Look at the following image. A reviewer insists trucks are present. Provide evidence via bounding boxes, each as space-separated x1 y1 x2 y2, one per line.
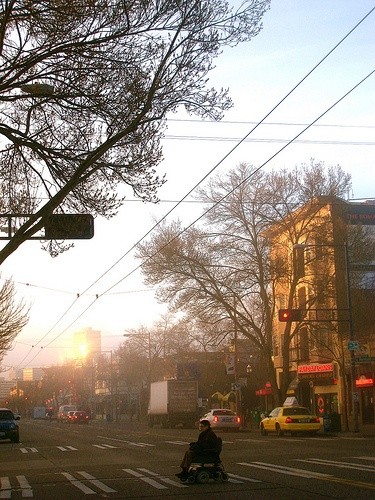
147 378 198 428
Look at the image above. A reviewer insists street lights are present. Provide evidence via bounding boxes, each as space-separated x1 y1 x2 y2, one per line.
13 84 54 94
91 350 114 422
123 333 151 385
198 293 241 416
294 241 361 433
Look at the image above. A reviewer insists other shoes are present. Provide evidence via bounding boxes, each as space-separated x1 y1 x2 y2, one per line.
176 470 190 478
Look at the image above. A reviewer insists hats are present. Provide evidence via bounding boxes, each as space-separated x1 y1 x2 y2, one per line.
200 420 210 426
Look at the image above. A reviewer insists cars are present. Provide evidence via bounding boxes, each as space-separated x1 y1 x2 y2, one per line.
260 397 321 437
0 408 20 443
33 404 89 425
200 409 241 432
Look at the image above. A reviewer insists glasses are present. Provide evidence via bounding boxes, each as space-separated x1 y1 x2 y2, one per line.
200 425 207 428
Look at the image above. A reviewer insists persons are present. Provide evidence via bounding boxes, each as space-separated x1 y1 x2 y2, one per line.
175 420 218 482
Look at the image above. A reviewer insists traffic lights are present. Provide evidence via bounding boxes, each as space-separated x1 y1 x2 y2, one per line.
278 309 302 322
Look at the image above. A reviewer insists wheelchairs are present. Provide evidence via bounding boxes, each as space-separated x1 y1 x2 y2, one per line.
174 437 229 484
